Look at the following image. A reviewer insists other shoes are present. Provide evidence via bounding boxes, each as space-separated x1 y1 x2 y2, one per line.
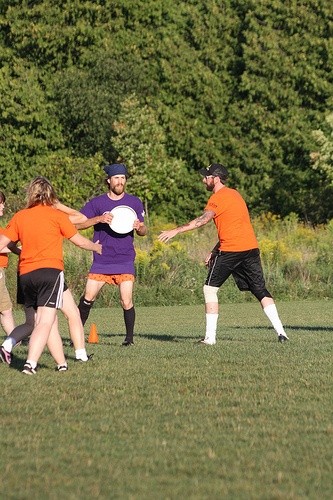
0 345 13 367
57 362 68 372
195 339 212 347
278 332 289 344
74 354 94 362
123 339 134 346
22 362 38 375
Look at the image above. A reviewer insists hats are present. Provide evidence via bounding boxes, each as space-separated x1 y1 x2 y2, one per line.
199 164 228 180
108 164 127 177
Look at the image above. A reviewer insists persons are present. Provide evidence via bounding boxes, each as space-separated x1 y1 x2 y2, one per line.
0 178 103 376
69 163 146 349
158 163 290 345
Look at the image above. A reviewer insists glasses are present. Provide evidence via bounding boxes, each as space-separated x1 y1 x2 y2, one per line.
205 175 215 179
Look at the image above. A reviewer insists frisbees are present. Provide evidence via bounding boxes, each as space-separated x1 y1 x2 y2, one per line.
107 204 138 235
